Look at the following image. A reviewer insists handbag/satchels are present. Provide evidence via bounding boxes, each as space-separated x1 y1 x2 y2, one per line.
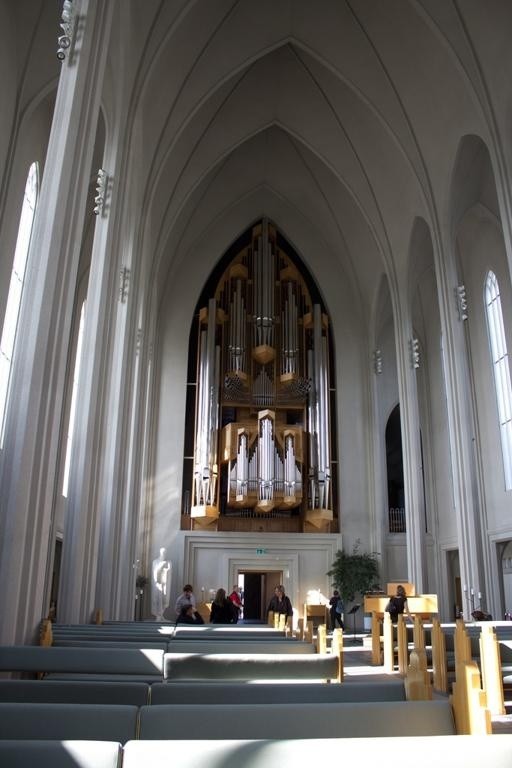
386 602 396 612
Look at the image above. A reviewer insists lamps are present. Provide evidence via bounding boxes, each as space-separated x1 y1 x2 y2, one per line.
90 169 108 216
55 1 74 62
411 337 422 369
454 283 469 322
191 221 335 530
373 348 383 376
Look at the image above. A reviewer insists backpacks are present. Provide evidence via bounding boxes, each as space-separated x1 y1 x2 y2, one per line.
336 599 344 614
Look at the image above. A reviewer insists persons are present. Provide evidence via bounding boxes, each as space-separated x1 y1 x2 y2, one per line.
329 590 345 633
174 584 244 625
385 585 415 627
267 585 293 621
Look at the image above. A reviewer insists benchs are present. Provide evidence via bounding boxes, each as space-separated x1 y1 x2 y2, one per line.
0 607 512 768
